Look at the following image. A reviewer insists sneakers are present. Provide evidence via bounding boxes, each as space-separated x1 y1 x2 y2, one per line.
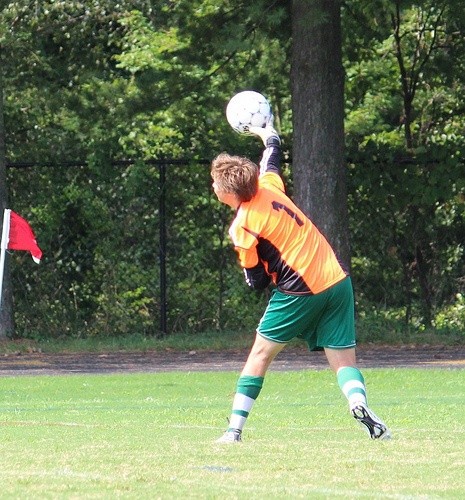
349 402 388 440
216 429 242 444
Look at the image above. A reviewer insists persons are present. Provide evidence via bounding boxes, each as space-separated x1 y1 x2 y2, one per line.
211 113 390 444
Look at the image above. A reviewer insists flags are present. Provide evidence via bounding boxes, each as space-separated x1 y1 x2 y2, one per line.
1 209 42 264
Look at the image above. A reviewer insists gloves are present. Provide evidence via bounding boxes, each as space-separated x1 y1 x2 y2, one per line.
249 114 283 146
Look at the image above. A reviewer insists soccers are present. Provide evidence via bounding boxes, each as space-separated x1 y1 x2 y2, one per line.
226 91 270 137
